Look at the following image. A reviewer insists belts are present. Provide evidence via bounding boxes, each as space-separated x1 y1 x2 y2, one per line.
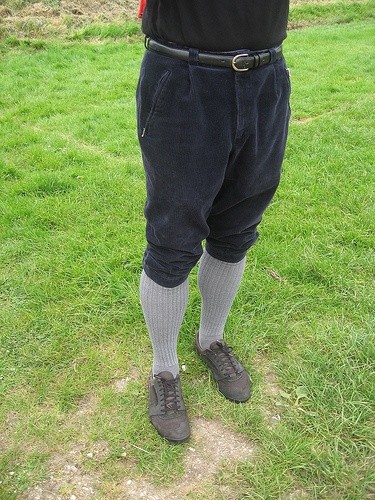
147 38 283 71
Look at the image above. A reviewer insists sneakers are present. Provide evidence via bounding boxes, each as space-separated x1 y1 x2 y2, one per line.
147 371 192 442
193 332 252 403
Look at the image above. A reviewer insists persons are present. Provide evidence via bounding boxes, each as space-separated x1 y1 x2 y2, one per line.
132 1 294 446
